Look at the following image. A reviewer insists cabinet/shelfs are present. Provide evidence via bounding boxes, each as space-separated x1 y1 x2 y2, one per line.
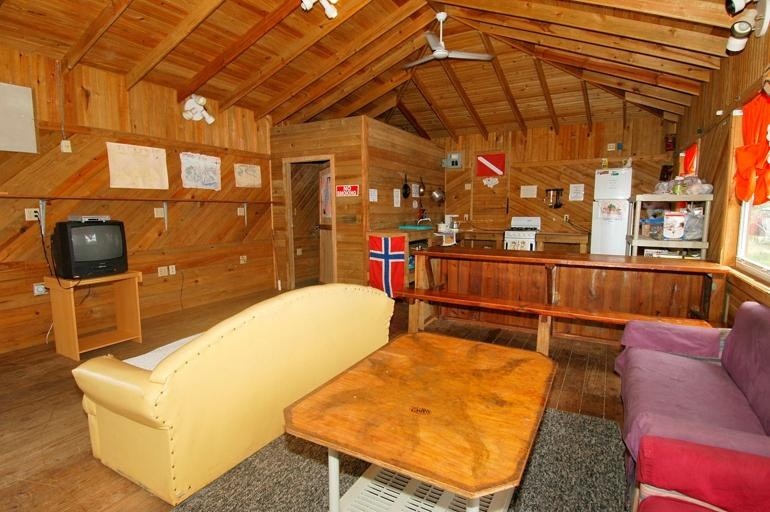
626 194 714 261
399 226 434 288
43 271 143 362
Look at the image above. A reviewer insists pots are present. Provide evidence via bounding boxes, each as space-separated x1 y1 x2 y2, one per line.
403 175 410 198
418 176 425 195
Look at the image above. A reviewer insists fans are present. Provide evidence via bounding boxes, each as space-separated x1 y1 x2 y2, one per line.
403 12 495 68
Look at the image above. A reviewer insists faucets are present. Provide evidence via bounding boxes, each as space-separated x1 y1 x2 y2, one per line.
417 218 431 224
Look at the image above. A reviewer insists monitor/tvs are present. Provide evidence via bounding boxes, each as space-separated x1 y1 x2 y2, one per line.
51 221 128 280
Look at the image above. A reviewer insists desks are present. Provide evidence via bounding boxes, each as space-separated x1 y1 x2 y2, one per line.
459 230 504 249
535 231 589 253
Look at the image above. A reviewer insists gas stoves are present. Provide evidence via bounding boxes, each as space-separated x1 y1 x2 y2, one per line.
505 227 537 231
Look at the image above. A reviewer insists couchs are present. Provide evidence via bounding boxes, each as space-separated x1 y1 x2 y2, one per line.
614 300 770 483
70 282 395 507
631 435 770 512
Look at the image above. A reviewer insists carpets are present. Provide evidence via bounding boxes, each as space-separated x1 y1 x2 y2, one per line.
168 407 629 512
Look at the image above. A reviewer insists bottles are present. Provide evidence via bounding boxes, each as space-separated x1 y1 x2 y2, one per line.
674 176 683 194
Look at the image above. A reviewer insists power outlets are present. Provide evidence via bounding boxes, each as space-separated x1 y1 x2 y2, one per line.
464 214 468 221
33 283 48 295
564 215 569 222
25 208 39 221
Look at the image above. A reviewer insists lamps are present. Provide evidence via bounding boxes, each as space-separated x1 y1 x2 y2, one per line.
725 0 770 56
301 0 338 19
182 93 215 124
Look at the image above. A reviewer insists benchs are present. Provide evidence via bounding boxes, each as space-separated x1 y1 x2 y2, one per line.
392 288 713 357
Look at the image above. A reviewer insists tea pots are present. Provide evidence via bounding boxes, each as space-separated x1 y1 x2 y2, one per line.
449 220 460 229
